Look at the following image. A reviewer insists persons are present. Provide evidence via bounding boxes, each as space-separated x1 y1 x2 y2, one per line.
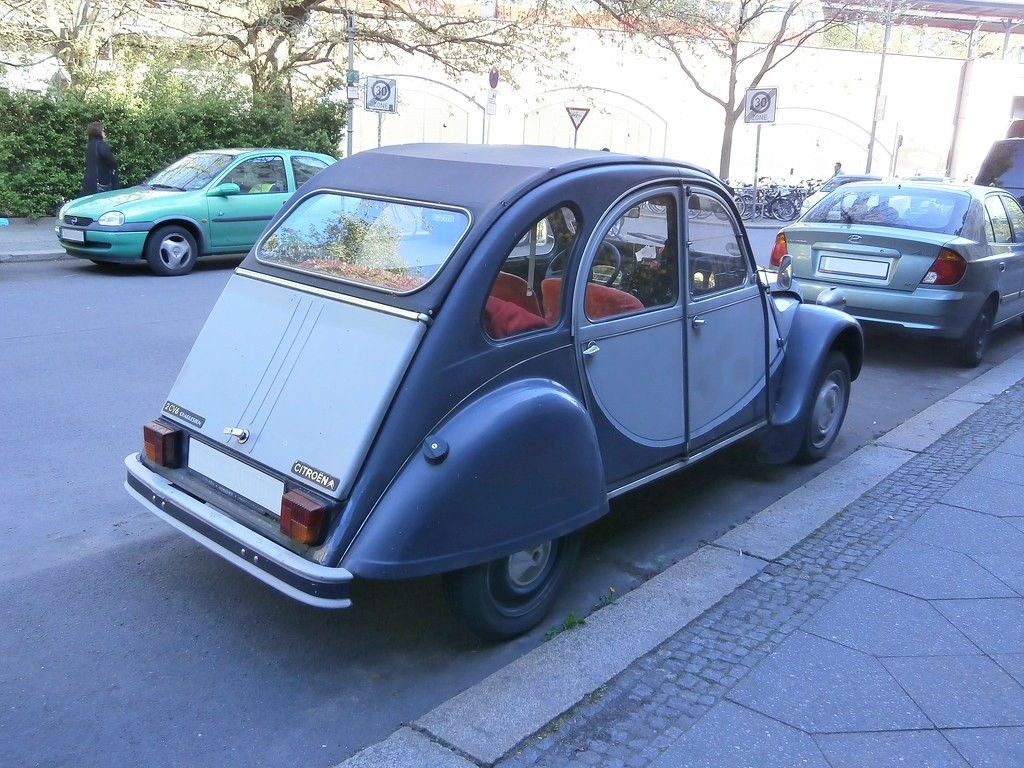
82 122 118 194
834 162 844 175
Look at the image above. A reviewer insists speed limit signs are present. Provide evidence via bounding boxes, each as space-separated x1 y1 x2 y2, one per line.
364 75 398 114
744 88 777 122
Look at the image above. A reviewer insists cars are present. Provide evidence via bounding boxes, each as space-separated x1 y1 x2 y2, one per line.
768 179 1024 368
53 148 338 276
124 141 865 639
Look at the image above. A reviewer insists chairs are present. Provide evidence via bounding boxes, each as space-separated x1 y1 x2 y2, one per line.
540 280 644 329
490 271 539 314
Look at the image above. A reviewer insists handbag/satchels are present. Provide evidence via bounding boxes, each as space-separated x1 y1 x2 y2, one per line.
96 182 113 193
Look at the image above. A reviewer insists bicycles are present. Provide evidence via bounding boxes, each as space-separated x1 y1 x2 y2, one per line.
640 177 824 222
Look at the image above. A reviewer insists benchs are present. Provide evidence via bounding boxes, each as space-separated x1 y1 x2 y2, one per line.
296 258 546 340
